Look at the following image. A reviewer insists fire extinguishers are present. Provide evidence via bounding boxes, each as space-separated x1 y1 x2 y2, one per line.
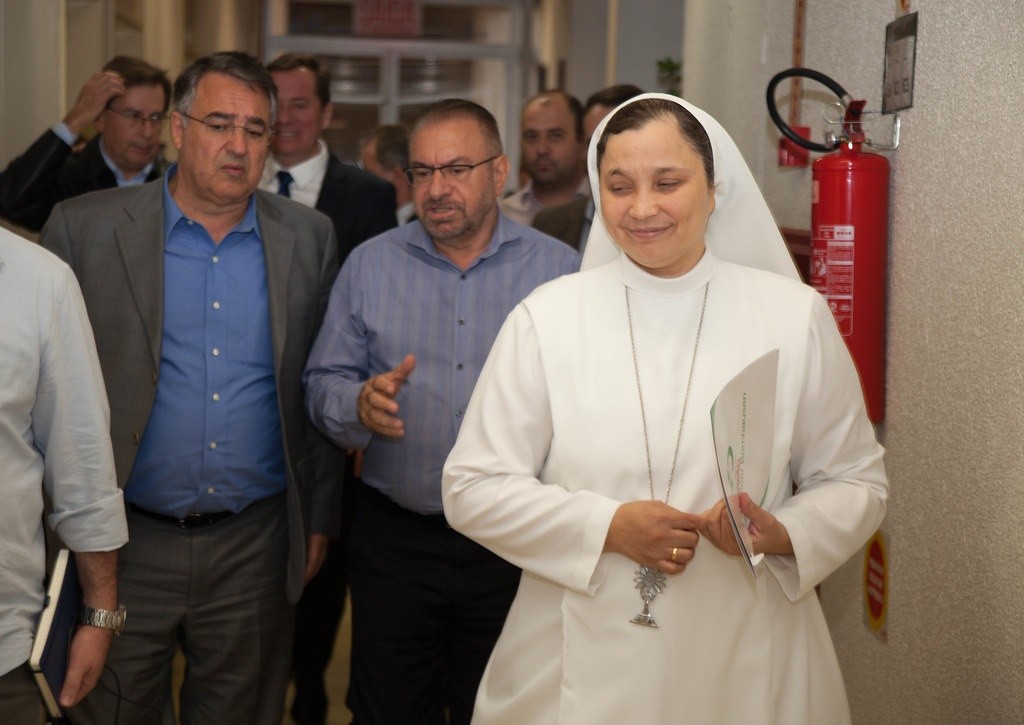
810 100 891 425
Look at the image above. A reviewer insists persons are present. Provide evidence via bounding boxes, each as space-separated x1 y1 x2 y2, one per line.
0 51 890 725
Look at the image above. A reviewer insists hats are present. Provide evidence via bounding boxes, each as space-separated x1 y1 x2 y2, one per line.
578 93 800 279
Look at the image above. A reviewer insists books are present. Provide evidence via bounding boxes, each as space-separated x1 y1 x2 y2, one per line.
28 550 82 719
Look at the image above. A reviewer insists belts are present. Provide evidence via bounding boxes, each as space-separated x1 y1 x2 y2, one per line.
125 498 261 531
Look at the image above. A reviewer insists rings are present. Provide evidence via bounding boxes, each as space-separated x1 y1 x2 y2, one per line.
669 547 678 561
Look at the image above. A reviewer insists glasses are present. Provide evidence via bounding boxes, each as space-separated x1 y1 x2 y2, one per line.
106 104 168 128
181 114 274 146
403 157 496 187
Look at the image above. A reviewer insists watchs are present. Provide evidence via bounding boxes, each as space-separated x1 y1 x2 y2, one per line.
78 604 127 636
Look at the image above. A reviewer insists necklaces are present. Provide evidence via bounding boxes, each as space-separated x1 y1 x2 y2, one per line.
624 281 709 630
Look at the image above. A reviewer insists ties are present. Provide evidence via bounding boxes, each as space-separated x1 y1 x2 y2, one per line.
277 170 293 197
